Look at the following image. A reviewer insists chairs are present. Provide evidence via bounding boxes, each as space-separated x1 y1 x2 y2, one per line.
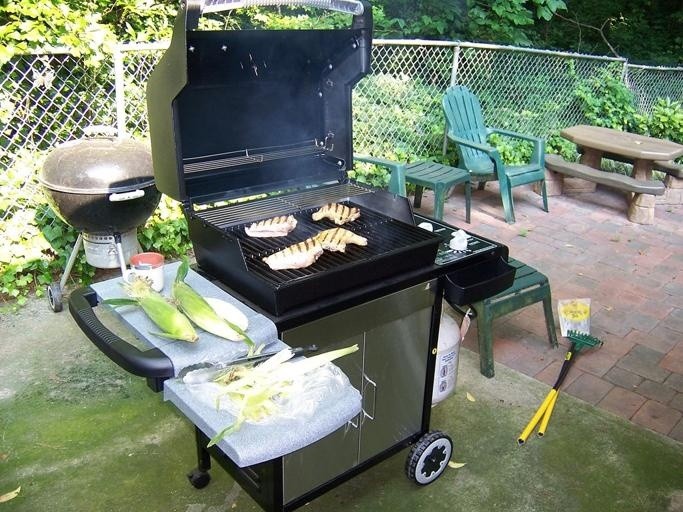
441 83 549 225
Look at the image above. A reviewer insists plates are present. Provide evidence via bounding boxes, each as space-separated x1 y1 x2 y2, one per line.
192 297 248 336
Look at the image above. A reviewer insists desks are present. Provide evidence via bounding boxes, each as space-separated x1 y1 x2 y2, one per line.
558 124 682 205
402 160 472 226
466 251 559 379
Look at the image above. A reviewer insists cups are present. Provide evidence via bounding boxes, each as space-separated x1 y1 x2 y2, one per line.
122 253 166 293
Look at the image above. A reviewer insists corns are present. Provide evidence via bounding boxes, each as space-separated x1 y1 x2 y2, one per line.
171 279 248 343
126 277 199 344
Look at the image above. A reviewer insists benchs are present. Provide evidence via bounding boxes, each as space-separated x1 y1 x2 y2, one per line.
543 145 683 226
